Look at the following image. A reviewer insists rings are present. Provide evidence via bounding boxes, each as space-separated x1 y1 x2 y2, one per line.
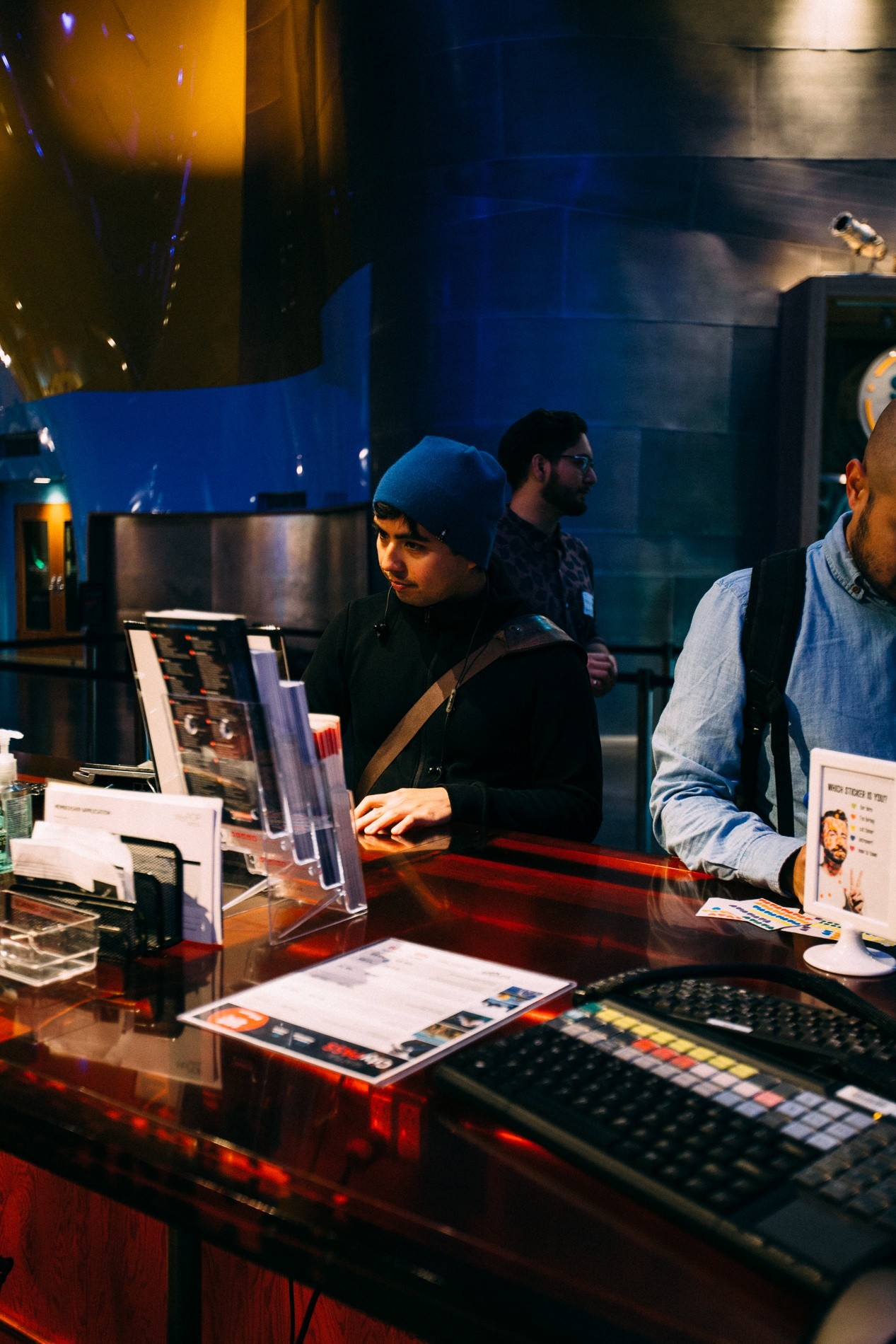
593 680 597 685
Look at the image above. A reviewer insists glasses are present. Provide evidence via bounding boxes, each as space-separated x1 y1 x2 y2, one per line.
561 452 594 471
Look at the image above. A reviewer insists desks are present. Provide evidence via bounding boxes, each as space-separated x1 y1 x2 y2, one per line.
0 763 896 1344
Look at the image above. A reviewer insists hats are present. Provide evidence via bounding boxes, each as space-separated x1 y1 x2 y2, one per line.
372 434 510 569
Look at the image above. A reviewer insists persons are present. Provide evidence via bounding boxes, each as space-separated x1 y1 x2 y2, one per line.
817 810 864 914
487 408 617 687
301 434 604 845
649 398 896 910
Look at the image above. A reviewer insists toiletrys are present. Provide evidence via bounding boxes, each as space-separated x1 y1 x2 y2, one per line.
0 728 34 873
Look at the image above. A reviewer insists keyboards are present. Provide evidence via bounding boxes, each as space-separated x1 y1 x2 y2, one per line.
434 993 896 1296
572 968 896 1098
